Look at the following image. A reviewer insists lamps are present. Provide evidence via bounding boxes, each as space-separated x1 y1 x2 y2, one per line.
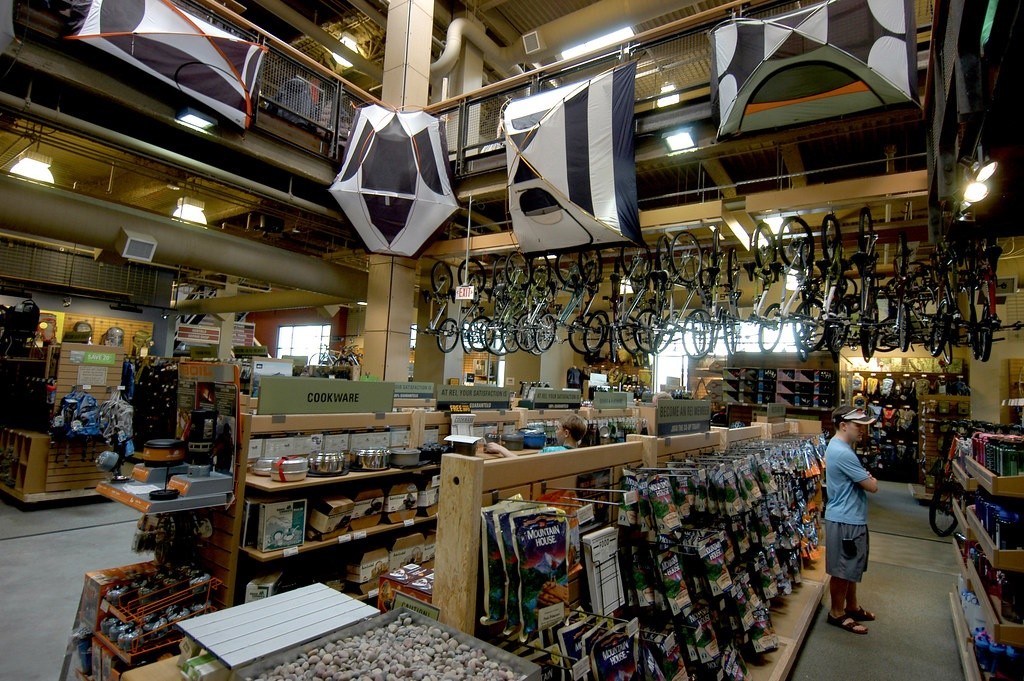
662 127 699 153
961 154 999 203
175 109 217 130
335 34 356 68
171 197 208 229
10 152 55 190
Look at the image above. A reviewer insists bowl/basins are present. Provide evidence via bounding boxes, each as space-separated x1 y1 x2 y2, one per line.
518 432 547 449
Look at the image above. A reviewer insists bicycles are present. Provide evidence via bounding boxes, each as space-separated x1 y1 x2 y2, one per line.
923 418 1023 537
420 207 1024 364
309 344 363 366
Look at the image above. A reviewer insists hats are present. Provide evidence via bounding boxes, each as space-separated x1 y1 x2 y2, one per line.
830 406 876 426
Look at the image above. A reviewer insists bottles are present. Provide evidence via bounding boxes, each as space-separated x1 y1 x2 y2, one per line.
543 418 648 447
974 491 1024 550
956 548 1024 681
972 433 1024 476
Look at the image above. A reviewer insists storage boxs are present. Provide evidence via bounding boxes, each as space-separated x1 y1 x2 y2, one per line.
256 498 306 549
73 560 159 681
241 496 283 547
389 532 423 569
422 531 436 562
347 548 390 583
244 570 284 604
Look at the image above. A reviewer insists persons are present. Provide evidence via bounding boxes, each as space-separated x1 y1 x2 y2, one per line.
486 414 586 457
825 406 875 633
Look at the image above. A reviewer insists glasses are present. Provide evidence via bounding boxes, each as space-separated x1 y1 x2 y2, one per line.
841 408 866 418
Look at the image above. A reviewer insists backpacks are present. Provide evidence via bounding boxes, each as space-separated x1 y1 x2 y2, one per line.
47 383 100 446
97 388 135 448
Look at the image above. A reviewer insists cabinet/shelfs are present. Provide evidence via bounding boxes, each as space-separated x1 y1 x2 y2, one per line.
208 398 832 681
0 429 51 493
687 356 972 483
950 455 1024 681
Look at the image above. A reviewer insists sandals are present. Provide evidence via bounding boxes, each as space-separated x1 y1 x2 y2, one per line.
827 609 869 635
843 606 876 622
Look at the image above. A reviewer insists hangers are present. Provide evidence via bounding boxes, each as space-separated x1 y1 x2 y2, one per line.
70 385 127 402
124 354 180 371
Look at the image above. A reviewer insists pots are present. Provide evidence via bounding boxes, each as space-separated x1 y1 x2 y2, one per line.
253 430 526 481
144 439 186 468
